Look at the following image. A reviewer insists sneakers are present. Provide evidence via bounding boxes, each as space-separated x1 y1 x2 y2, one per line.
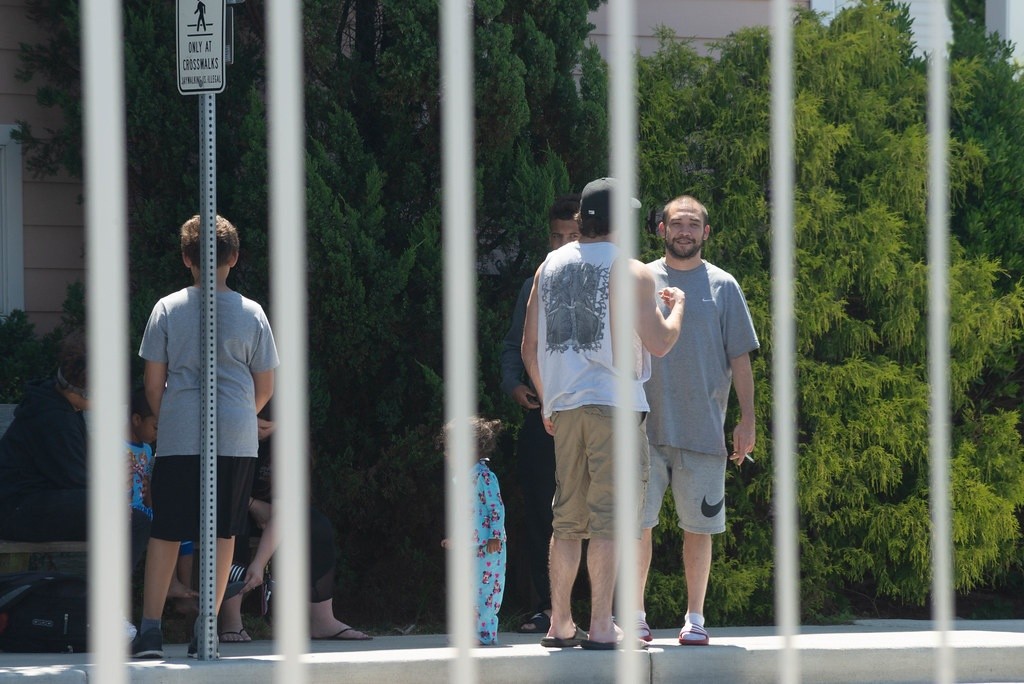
187 635 221 659
128 628 165 659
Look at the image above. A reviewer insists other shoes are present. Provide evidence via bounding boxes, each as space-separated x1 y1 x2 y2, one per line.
127 622 137 644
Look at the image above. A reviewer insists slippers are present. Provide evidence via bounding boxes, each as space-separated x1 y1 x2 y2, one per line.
518 612 550 633
678 626 709 646
579 635 650 652
220 579 245 602
311 628 374 640
219 628 252 643
635 621 653 641
539 623 590 648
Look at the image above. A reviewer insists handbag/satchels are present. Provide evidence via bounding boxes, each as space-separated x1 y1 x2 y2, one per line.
241 580 272 619
0 569 119 654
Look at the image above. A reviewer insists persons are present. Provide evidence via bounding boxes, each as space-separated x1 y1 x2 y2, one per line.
521 178 686 651
435 417 507 647
502 196 582 636
122 399 197 616
218 401 372 642
634 196 756 645
139 212 279 658
0 326 137 650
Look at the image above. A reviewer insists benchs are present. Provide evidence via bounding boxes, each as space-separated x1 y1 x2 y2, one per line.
0 539 88 578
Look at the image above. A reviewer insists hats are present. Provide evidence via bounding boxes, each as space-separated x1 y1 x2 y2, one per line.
580 176 643 219
